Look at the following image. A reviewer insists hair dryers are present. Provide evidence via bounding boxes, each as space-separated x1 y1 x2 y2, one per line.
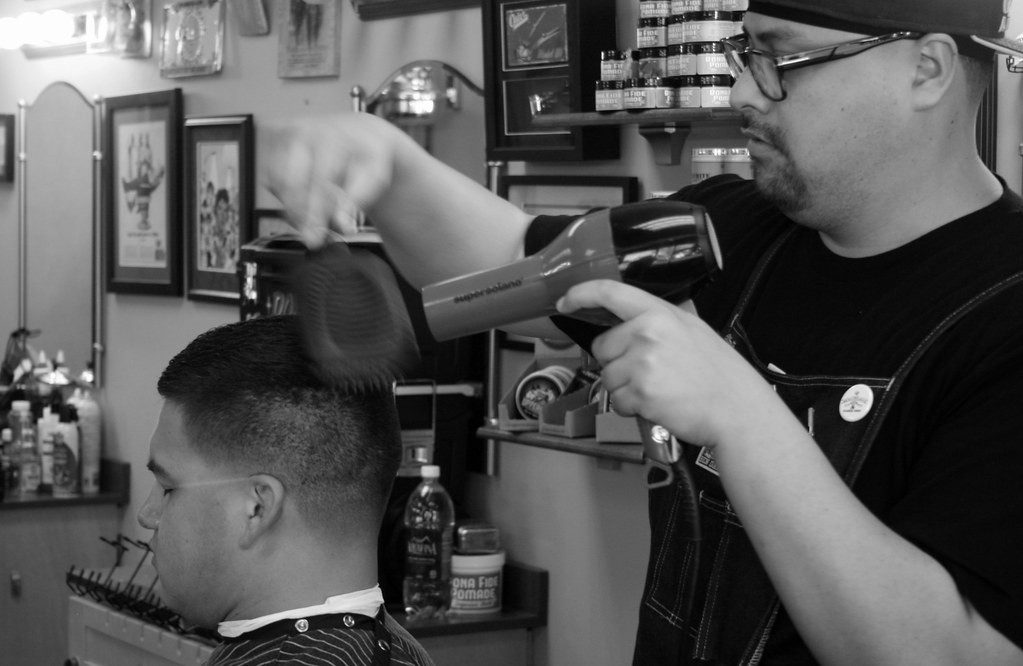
420 197 726 466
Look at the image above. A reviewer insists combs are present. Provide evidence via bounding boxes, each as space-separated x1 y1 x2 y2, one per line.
294 226 421 395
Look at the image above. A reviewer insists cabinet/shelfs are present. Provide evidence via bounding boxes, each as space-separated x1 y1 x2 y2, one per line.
478 161 645 475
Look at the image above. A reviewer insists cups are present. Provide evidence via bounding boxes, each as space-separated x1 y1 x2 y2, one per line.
515 365 575 420
450 549 505 614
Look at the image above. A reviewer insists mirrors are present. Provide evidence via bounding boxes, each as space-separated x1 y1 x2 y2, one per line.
349 61 488 235
16 81 101 388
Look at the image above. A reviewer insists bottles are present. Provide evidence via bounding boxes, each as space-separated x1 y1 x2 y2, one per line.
691 147 752 185
0 385 101 498
402 464 454 618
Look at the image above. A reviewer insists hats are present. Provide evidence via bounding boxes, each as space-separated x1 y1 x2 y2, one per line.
747 0 1004 62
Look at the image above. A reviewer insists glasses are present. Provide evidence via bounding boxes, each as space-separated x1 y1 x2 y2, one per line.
720 31 920 102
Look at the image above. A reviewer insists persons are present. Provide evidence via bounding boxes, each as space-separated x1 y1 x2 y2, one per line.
262 4 1023 666
137 317 441 665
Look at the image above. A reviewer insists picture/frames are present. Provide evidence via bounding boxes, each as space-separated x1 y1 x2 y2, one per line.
186 115 253 305
105 88 187 296
